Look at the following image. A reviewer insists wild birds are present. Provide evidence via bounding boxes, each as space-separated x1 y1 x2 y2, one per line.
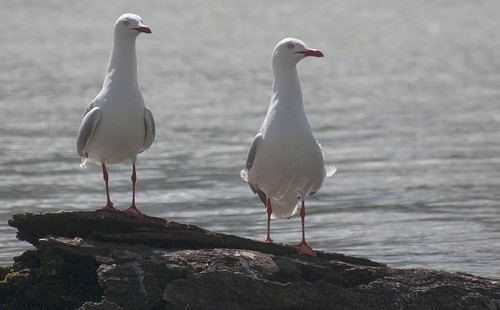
240 37 337 256
77 13 155 220
